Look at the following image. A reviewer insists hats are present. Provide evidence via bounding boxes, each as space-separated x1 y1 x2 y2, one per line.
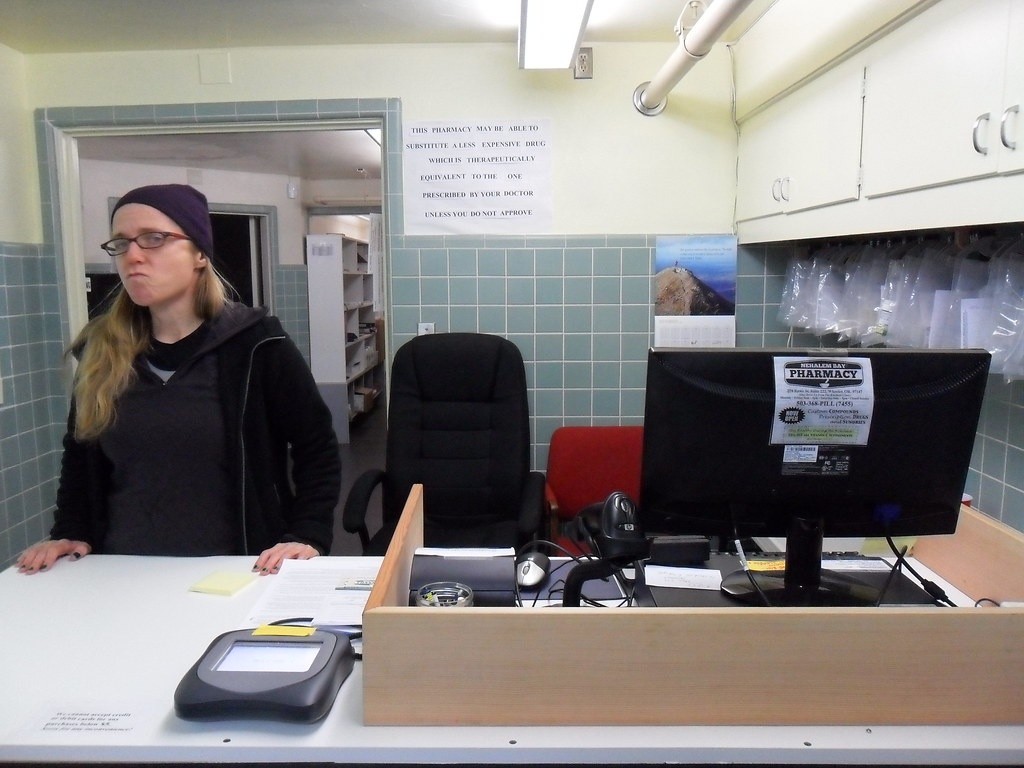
111 184 214 262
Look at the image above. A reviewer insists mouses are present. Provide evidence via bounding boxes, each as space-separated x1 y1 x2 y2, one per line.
515 552 552 587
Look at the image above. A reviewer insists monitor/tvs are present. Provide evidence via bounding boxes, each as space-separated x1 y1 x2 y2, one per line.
634 347 993 609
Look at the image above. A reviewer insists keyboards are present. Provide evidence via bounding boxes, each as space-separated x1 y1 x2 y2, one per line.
710 548 864 559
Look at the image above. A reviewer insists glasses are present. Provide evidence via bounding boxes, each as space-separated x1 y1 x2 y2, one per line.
99 231 191 256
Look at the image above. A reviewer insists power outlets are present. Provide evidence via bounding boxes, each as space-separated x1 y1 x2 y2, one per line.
573 47 593 78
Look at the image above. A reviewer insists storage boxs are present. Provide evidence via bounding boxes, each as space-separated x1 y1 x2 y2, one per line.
354 388 373 412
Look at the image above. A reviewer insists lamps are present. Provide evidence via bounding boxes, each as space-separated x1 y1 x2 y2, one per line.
517 0 593 70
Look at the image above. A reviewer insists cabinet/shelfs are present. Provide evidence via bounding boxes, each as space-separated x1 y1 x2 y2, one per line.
864 0 1024 198
306 234 381 443
735 48 863 223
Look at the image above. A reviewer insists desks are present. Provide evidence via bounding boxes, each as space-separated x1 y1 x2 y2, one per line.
1 555 1024 768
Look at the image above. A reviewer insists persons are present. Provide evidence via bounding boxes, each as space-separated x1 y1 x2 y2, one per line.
14 184 340 577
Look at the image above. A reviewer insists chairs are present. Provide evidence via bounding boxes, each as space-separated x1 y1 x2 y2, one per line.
343 333 551 556
545 426 644 557
